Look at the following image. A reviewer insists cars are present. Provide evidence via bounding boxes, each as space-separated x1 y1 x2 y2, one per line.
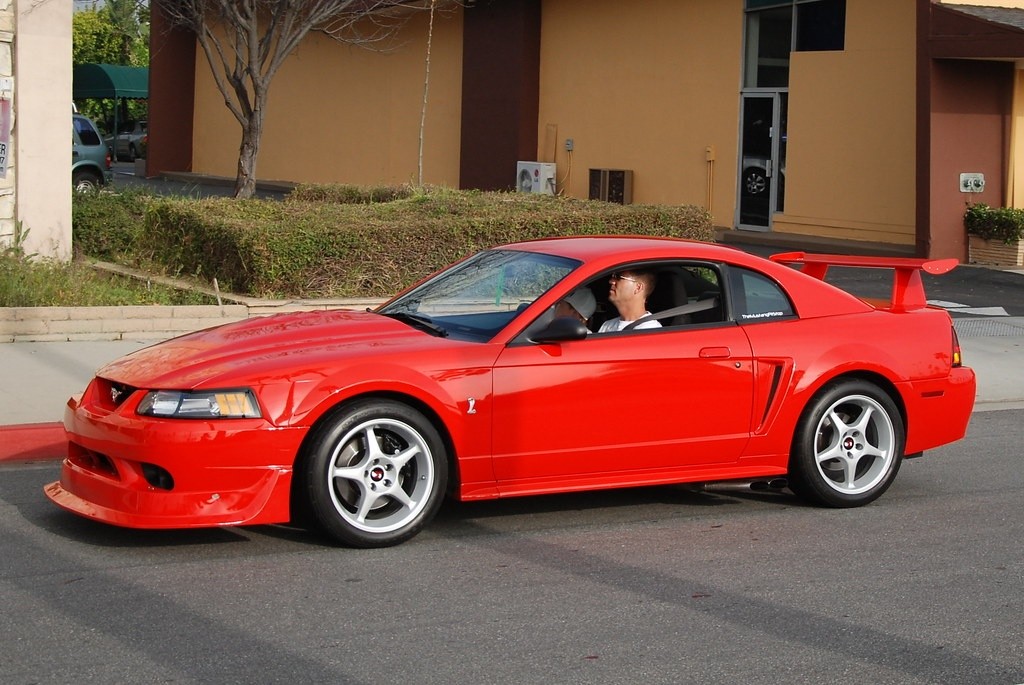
103 120 148 163
740 131 785 214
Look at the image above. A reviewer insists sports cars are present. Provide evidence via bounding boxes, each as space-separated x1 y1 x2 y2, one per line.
48 235 977 549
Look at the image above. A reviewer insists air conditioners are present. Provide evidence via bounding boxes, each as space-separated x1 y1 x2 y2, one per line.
516 161 557 195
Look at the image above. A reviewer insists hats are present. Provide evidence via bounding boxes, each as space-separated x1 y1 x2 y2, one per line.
563 287 596 320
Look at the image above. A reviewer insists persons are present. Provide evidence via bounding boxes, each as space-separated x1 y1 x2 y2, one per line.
598 269 661 332
551 287 596 335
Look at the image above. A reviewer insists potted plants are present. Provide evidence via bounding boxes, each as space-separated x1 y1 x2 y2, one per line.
964 201 1024 265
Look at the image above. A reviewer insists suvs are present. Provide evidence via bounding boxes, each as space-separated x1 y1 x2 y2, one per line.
71 112 113 200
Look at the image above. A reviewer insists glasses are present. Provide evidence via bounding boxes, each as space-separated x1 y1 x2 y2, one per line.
610 273 638 283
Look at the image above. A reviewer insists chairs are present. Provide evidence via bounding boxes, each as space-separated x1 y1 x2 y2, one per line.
651 265 688 327
